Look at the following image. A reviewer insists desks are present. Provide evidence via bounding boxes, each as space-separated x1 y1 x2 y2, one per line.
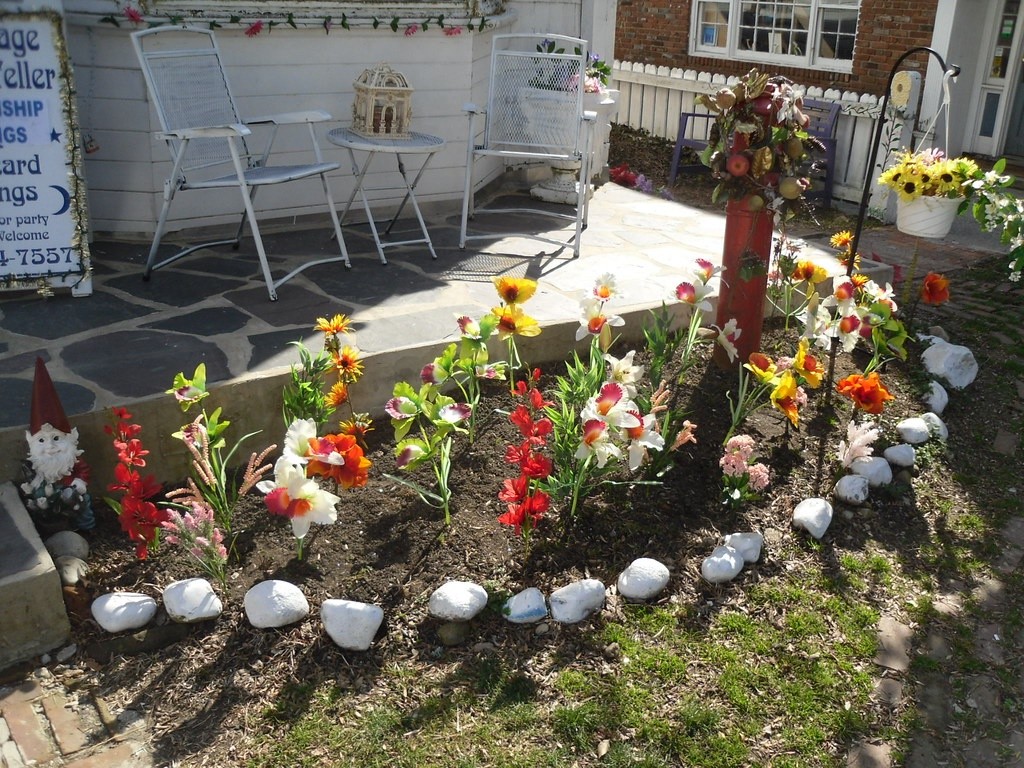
327 127 446 265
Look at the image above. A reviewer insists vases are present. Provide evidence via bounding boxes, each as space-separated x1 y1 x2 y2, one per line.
896 190 966 239
520 86 619 205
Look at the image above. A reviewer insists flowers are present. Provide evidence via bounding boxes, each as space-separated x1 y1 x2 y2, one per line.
877 147 1024 282
101 230 950 594
246 21 264 38
443 24 463 38
528 39 611 94
404 23 418 35
122 5 143 25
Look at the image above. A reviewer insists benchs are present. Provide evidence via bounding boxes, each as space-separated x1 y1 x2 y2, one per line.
668 98 842 212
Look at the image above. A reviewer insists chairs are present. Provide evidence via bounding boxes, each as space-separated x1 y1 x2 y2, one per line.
460 32 597 258
131 25 352 302
